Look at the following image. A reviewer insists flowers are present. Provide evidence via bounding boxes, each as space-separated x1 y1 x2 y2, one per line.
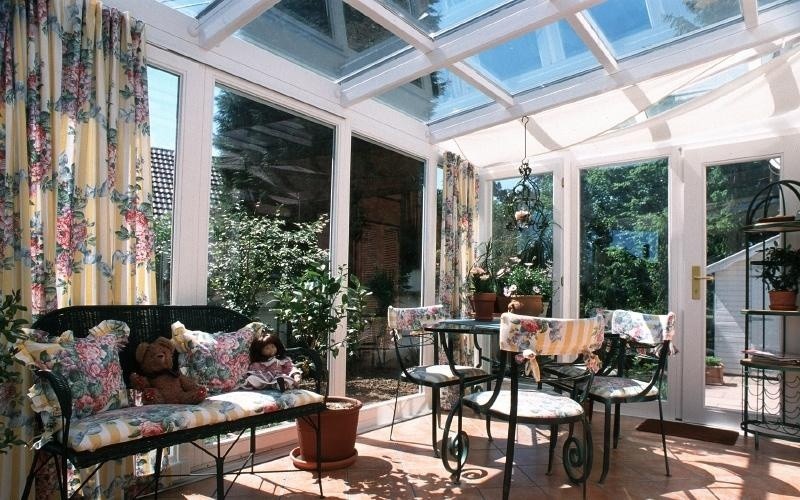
467 243 497 293
499 256 560 301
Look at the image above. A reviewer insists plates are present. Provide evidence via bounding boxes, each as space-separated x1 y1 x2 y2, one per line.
289 447 359 471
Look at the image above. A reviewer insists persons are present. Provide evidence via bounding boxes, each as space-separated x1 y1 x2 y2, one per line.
239 333 303 392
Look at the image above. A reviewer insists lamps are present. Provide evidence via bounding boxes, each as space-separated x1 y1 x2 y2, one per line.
507 116 547 233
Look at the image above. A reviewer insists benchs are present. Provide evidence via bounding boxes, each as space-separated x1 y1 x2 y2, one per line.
19 305 324 500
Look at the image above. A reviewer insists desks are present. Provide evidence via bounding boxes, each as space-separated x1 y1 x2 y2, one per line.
423 317 621 484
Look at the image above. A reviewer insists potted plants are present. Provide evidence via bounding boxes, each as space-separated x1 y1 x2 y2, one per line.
751 239 800 312
706 354 726 385
265 265 375 465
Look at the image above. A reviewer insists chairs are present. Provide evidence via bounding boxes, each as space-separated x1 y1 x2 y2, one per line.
386 305 499 457
537 307 626 449
544 309 675 481
441 311 605 500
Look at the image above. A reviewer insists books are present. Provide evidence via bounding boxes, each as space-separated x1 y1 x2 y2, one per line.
739 349 800 368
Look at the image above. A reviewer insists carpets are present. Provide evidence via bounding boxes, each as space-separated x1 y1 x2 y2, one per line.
634 417 741 447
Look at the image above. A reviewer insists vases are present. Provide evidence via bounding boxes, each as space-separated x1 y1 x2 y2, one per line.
508 294 545 317
471 291 497 322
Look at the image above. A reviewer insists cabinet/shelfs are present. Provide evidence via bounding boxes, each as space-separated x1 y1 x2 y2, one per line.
740 179 800 451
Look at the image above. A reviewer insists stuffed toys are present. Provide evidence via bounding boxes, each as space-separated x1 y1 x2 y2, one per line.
129 336 208 406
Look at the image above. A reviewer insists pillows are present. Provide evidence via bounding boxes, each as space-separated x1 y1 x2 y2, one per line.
170 320 268 397
9 315 137 452
